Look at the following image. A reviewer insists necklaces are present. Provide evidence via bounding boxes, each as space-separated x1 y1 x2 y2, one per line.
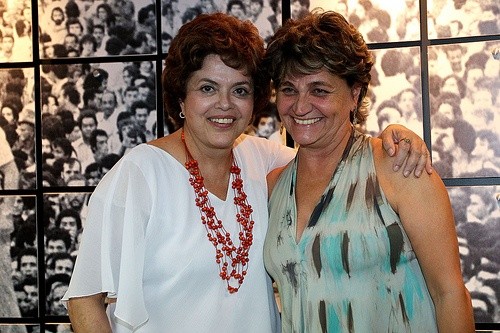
182 121 255 294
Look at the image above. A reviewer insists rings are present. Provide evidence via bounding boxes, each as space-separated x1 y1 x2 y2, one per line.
399 137 412 147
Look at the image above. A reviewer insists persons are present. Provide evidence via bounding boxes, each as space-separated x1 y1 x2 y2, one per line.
0 1 500 323
0 129 27 333
67 13 433 333
260 8 475 333
